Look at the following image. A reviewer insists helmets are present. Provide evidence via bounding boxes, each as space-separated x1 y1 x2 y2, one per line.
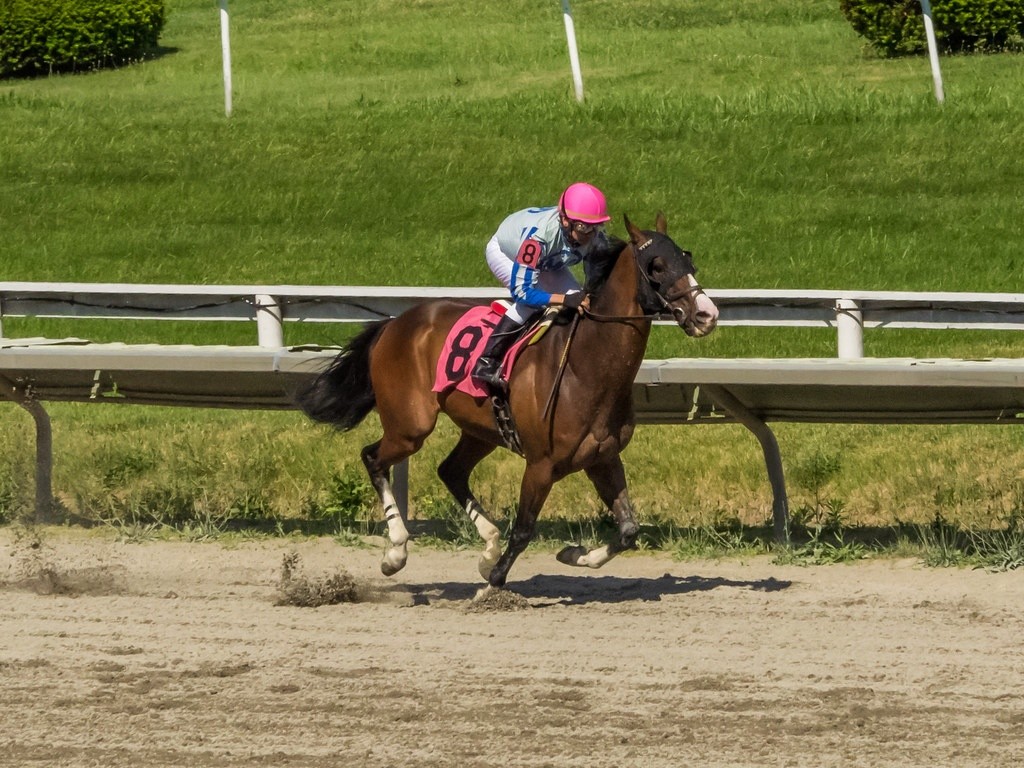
557 182 610 223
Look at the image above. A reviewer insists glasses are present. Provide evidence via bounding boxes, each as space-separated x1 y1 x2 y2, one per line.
562 183 605 235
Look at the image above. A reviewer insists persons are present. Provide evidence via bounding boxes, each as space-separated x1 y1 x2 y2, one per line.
469 182 612 388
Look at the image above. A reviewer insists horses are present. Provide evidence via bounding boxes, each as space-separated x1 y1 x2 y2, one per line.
293 207 721 589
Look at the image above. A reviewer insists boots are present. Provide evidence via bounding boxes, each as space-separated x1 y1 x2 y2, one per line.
470 314 524 384
